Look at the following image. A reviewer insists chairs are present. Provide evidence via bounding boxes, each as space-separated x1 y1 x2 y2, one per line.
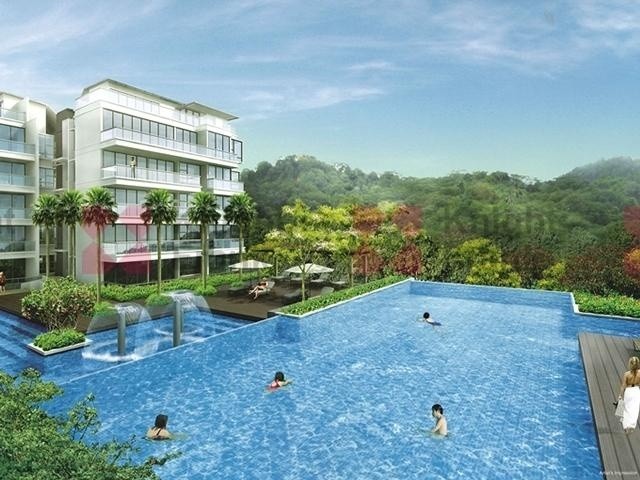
249 280 274 300
283 287 308 304
309 286 335 301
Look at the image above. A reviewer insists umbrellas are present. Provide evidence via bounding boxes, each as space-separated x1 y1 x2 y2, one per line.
227 259 274 284
283 262 335 298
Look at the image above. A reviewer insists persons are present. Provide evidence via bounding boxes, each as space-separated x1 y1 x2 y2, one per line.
268 371 296 391
421 403 449 436
248 276 268 300
420 311 446 330
612 356 639 435
146 414 174 441
